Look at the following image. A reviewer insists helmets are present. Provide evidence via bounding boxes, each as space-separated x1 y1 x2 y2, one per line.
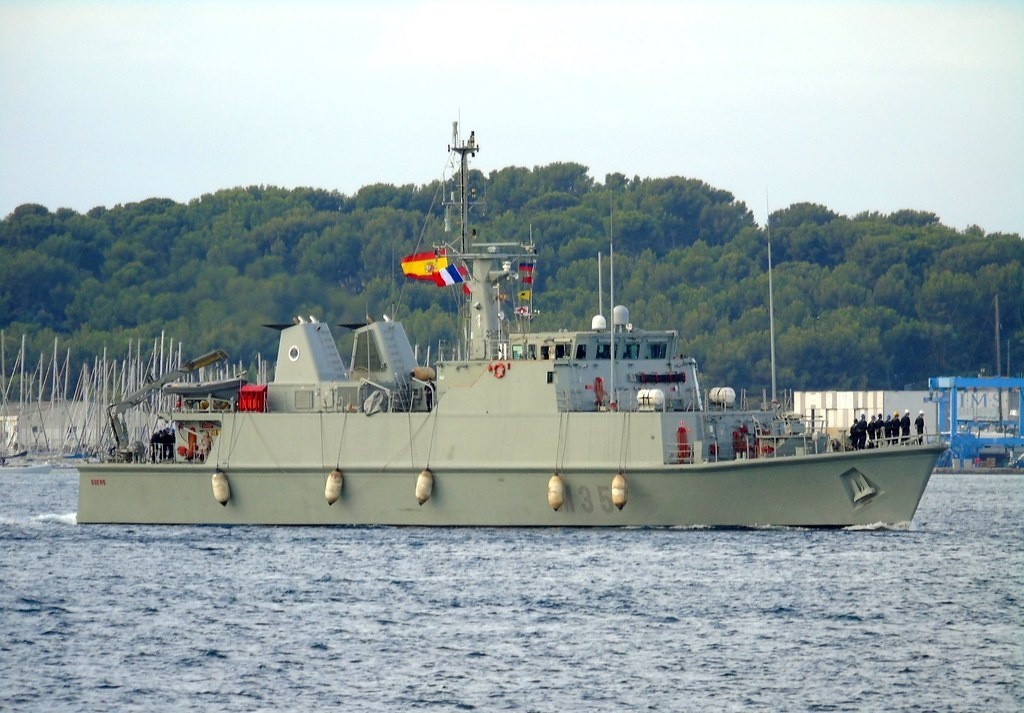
893 411 899 416
861 414 866 418
887 415 890 419
905 409 909 414
919 410 925 414
871 416 875 420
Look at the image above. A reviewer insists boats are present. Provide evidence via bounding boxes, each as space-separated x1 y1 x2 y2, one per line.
76 116 956 527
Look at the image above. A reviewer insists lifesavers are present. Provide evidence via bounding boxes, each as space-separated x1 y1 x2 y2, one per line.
494 363 505 378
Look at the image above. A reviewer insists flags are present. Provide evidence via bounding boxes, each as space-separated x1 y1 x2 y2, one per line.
517 290 530 301
432 261 464 287
401 249 448 281
523 276 531 283
519 262 533 272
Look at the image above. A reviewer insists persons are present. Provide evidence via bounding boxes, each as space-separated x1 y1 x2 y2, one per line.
850 409 925 450
151 429 173 463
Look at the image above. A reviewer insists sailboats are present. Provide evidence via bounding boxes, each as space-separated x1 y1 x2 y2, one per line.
1 331 241 469
